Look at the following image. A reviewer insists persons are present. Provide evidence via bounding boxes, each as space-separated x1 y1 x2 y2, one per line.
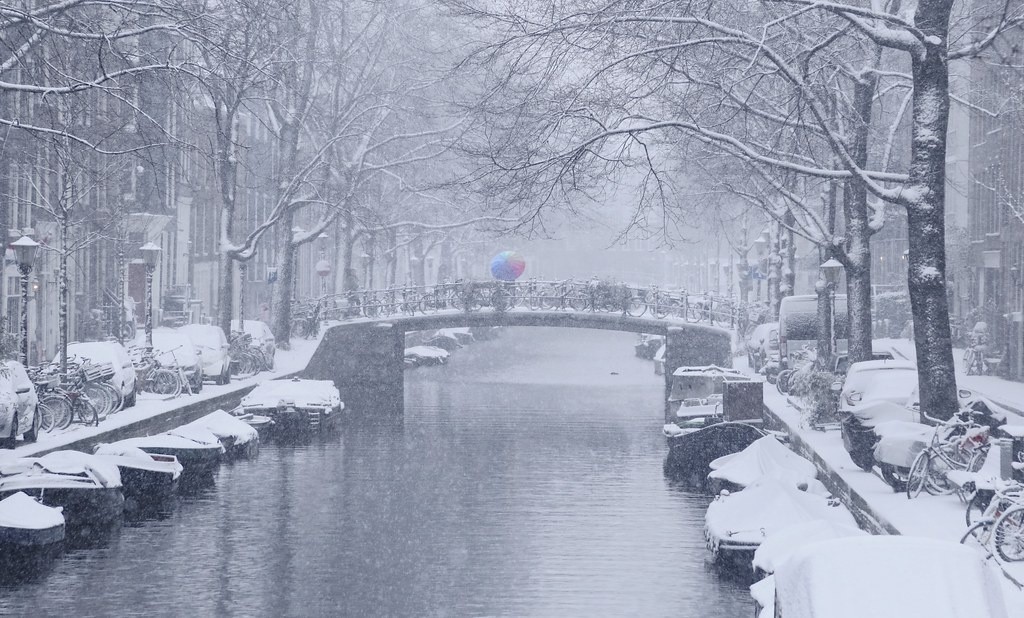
344 269 362 317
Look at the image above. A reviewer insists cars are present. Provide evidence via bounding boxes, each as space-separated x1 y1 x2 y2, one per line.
176 323 233 387
231 318 275 371
1 358 42 451
230 318 277 370
138 327 204 395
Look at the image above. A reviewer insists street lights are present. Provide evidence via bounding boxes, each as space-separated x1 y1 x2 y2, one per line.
755 237 766 309
138 240 164 361
360 253 370 290
317 231 330 296
9 236 42 377
818 252 846 370
290 226 306 298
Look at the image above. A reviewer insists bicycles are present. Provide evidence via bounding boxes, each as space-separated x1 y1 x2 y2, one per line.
292 277 1024 562
25 333 274 434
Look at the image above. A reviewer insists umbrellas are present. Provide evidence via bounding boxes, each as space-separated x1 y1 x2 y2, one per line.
491 251 525 280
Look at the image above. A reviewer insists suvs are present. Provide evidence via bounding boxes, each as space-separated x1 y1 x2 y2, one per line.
50 340 138 409
